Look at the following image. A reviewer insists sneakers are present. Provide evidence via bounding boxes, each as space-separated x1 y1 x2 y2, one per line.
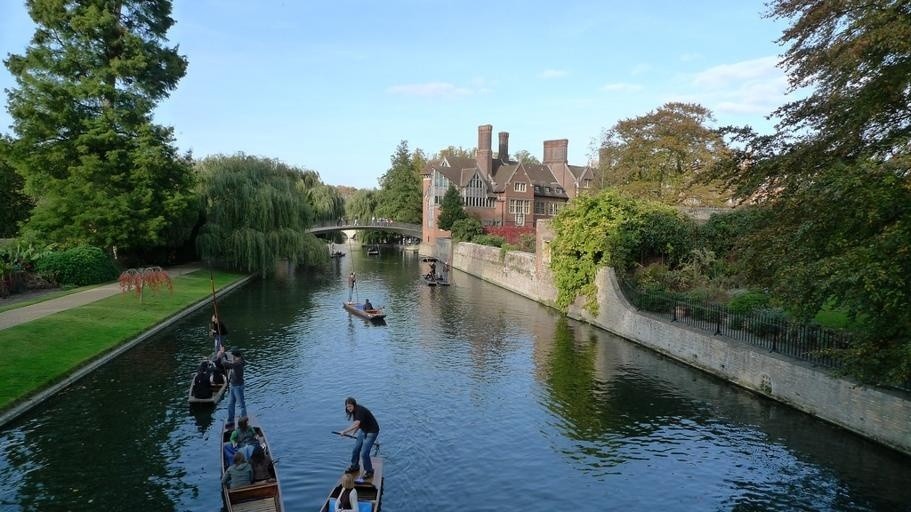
345 466 359 473
360 469 373 479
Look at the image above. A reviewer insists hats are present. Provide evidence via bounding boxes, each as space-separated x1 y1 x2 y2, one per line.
238 416 248 426
231 351 242 357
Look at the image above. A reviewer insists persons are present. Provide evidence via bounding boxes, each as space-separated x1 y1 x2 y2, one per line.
364 298 373 312
430 260 437 282
334 473 359 510
336 213 395 227
194 343 228 399
210 313 228 356
339 396 382 478
348 271 356 306
443 259 450 282
220 344 248 433
220 413 271 490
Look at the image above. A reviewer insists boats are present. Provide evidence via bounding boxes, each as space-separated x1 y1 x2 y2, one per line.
320 454 382 512
188 350 233 404
330 252 345 259
342 301 386 320
422 274 450 286
221 415 284 511
